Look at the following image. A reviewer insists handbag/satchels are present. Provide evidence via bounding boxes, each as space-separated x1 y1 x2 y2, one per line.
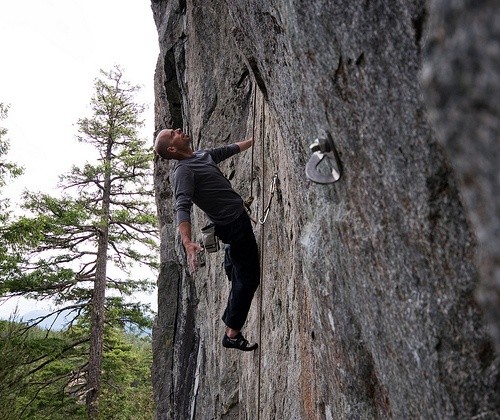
199 223 220 253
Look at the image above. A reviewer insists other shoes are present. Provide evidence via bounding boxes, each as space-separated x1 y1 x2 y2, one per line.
221 331 258 351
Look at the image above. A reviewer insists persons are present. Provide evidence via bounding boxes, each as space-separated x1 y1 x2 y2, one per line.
154 127 260 351
